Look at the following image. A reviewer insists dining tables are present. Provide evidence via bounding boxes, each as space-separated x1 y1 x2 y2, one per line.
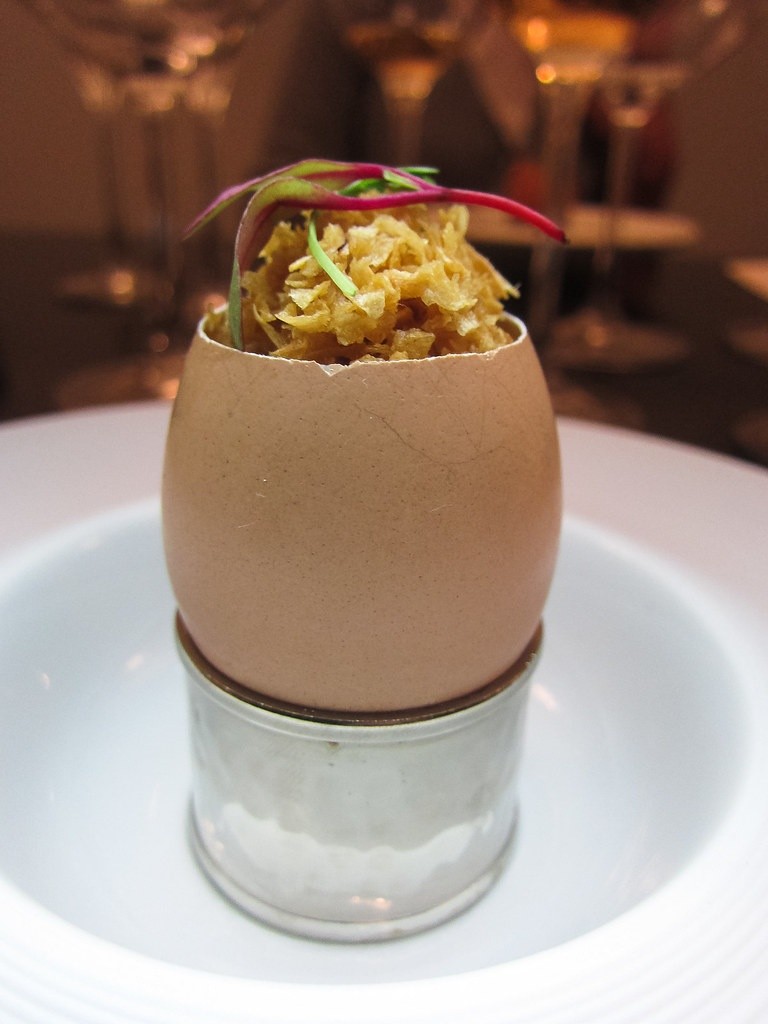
0 401 768 1010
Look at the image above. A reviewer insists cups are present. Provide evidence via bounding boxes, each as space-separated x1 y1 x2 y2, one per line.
176 609 544 944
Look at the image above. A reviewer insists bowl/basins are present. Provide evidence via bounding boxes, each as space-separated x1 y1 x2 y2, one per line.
0 402 768 1024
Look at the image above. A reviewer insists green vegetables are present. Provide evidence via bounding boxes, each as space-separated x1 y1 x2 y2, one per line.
304 164 443 312
